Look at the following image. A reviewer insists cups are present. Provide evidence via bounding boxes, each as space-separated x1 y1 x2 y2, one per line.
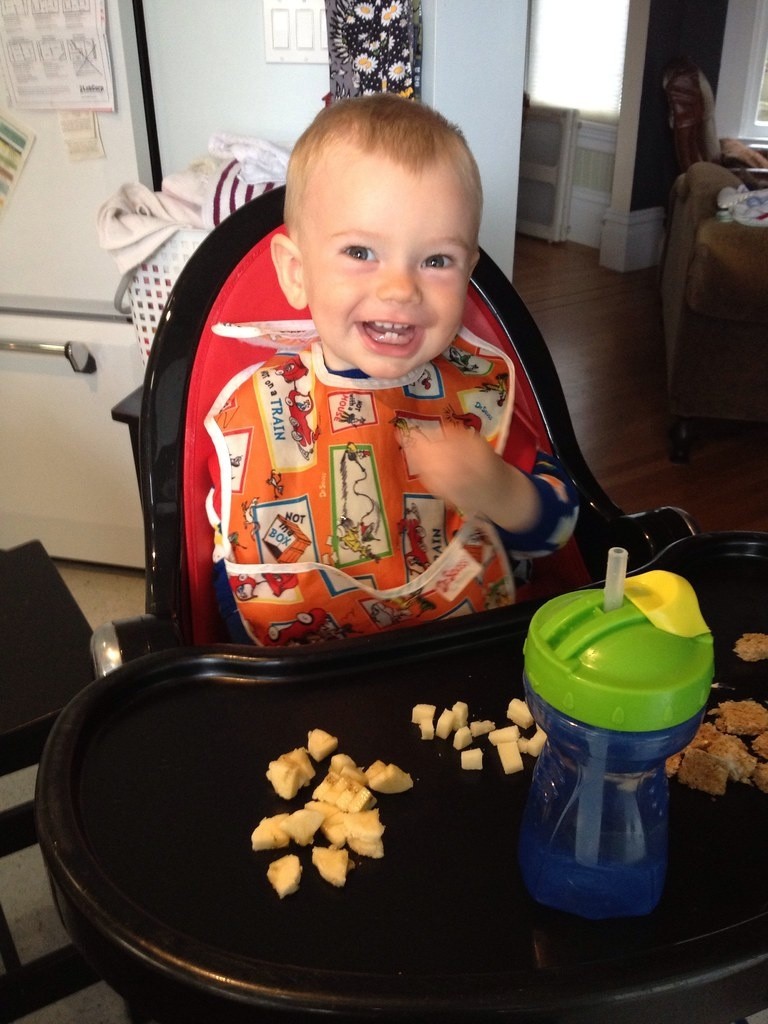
522 571 713 918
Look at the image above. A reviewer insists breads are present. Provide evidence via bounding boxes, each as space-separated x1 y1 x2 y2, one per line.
661 633 768 795
411 698 549 775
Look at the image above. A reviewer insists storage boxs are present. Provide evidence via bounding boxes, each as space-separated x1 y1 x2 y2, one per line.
127 226 209 367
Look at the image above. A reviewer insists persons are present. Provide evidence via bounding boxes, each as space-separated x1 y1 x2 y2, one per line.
204 97 579 646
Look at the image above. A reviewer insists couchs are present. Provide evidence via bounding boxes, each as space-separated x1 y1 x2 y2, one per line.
657 161 768 467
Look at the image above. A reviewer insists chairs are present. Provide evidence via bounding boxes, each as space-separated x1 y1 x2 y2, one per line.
0 538 107 1024
89 184 703 690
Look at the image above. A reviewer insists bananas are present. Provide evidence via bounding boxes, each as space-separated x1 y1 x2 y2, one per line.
251 729 413 896
